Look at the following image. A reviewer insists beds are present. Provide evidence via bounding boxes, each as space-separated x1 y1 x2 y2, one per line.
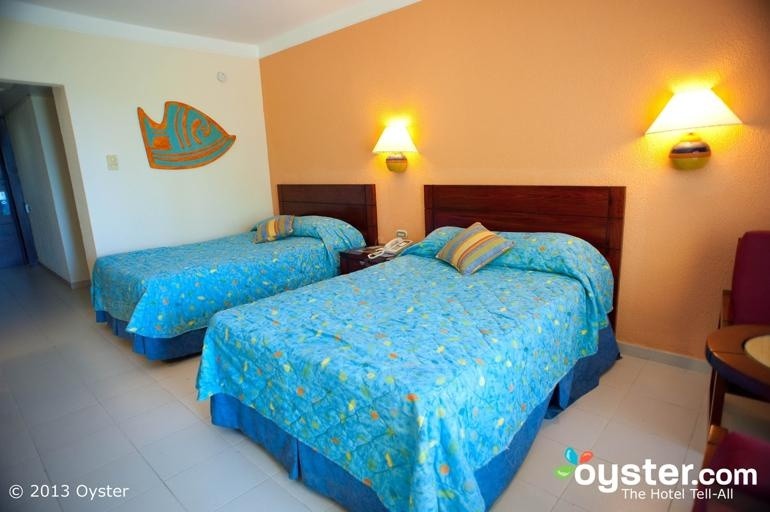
87 183 627 511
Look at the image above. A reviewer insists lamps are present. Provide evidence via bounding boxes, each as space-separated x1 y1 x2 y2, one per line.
372 123 417 172
643 88 743 171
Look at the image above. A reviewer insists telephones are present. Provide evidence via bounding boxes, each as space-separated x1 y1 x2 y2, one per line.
384 238 413 256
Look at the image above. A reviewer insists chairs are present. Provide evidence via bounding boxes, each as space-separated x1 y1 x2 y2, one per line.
693 229 768 510
